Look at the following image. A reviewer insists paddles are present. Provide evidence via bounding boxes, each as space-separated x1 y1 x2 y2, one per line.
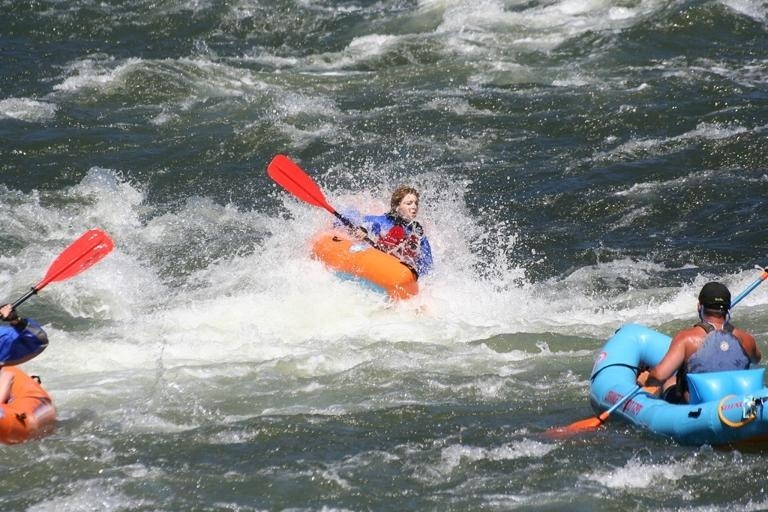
0 228 114 319
267 154 376 248
546 270 768 436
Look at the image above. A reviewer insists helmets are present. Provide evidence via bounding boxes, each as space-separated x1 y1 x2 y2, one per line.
698 282 731 310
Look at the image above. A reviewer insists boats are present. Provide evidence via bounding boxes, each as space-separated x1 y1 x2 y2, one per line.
0 365 57 445
311 229 418 301
588 324 768 444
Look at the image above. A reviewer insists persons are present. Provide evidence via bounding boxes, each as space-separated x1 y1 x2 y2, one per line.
353 187 433 275
636 281 761 404
0 303 49 365
